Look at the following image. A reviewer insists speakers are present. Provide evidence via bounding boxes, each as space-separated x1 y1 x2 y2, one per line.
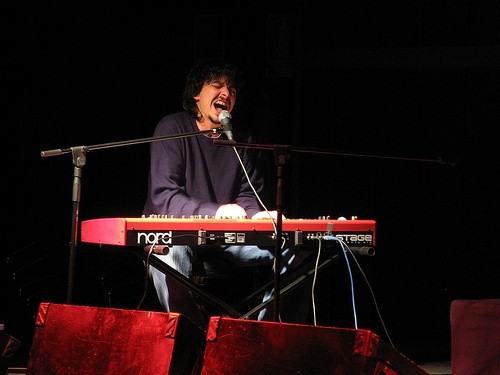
200 316 431 375
25 302 206 375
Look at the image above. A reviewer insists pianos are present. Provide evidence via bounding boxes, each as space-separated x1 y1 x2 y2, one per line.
81 214 376 246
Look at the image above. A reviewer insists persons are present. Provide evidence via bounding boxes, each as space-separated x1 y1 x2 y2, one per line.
139 59 295 321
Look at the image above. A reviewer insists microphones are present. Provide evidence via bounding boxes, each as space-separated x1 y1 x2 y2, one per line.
217 110 233 141
